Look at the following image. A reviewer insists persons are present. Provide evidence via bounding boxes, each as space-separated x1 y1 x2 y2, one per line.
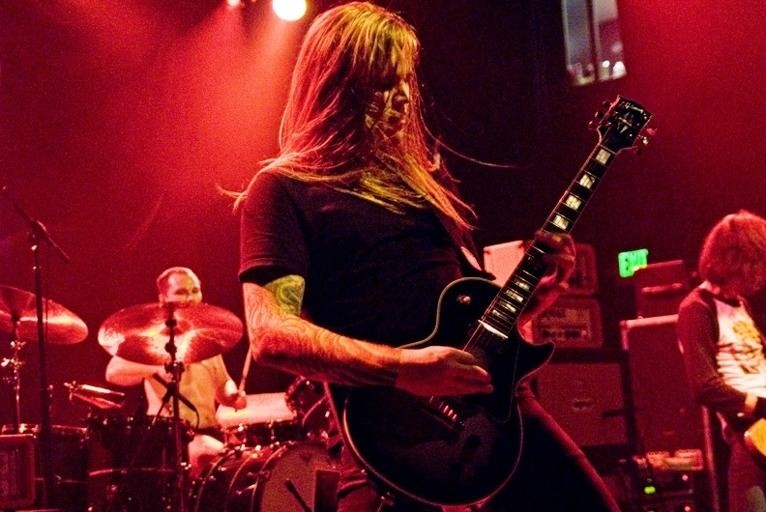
106 268 247 442
675 208 766 512
230 1 624 512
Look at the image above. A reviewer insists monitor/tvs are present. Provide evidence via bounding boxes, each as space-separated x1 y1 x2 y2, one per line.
610 240 658 286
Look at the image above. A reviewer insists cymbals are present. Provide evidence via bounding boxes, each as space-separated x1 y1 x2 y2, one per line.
0 282 90 347
95 300 245 367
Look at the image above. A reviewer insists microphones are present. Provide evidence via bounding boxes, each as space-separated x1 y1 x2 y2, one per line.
152 373 197 412
45 234 73 264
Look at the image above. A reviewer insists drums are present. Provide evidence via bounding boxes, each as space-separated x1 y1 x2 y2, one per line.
225 419 300 447
85 466 185 512
2 421 92 480
190 440 337 512
90 413 194 472
283 373 343 452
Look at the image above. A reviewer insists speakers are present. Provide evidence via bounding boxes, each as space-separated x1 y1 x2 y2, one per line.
618 312 723 512
531 348 637 462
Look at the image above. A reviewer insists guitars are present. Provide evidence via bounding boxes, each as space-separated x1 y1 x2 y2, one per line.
341 94 659 510
745 416 766 463
